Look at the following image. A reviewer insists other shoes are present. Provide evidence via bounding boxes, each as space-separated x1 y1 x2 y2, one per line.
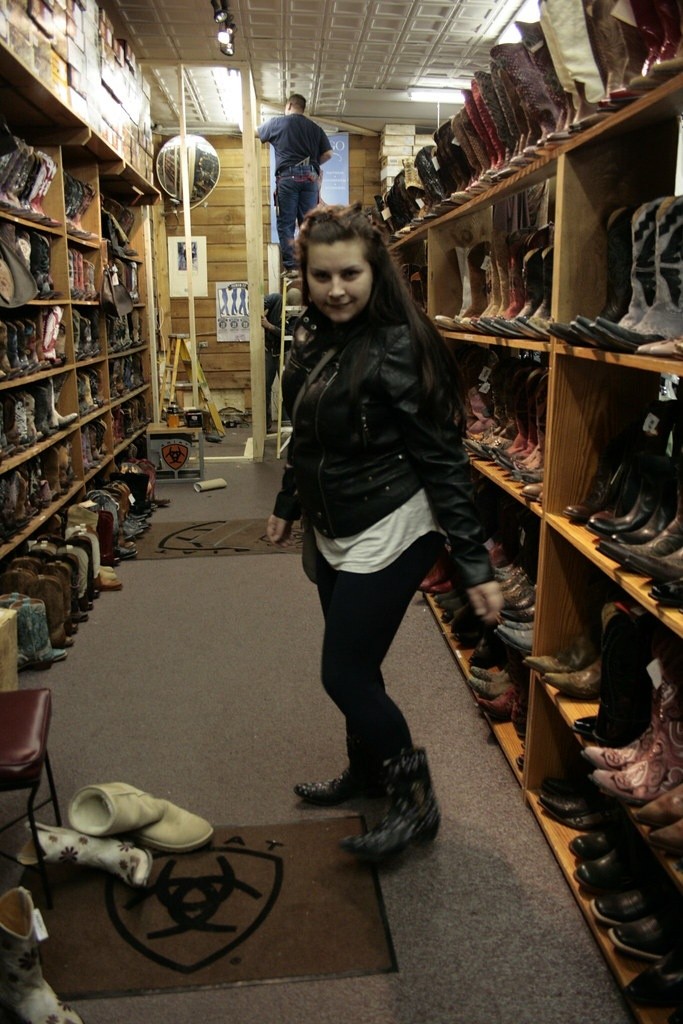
416 539 683 1024
280 269 299 279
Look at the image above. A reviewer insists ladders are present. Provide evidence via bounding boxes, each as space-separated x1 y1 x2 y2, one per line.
277 275 305 460
159 333 226 437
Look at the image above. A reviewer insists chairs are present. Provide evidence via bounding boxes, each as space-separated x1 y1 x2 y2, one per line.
1 686 64 910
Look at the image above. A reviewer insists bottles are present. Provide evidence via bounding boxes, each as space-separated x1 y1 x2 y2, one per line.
166 402 179 429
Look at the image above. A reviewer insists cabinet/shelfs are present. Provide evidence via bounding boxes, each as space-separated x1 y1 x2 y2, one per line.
391 68 683 1024
147 422 205 483
0 38 161 683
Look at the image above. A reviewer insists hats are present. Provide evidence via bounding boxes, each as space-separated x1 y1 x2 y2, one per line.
285 287 302 305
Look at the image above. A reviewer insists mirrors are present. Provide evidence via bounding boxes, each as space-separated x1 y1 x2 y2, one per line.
156 134 220 202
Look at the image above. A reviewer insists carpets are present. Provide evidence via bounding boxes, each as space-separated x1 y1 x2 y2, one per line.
128 521 303 560
19 816 401 999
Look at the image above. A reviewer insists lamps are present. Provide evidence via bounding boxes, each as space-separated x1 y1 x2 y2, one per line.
219 33 234 56
217 16 237 44
211 0 227 23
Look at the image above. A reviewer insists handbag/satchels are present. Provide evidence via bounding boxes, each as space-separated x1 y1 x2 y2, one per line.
300 516 326 583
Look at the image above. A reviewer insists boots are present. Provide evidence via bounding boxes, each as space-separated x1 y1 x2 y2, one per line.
66 782 215 853
335 745 440 855
15 821 153 888
0 886 85 1024
0 120 172 672
292 763 362 806
362 0 683 854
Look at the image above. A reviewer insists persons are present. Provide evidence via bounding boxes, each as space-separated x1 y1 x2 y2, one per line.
254 94 334 278
261 288 303 438
267 204 505 862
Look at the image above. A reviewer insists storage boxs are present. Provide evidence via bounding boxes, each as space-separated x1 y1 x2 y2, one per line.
379 122 438 195
0 0 155 183
